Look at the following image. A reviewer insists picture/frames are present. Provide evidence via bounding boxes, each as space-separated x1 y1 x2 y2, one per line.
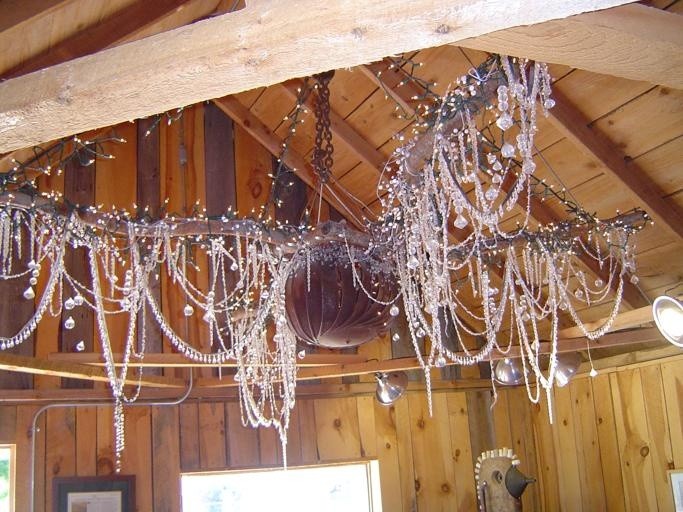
51 475 135 512
666 469 683 512
0 443 16 512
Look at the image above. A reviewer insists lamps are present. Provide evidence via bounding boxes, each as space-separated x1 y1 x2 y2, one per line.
652 282 683 348
374 370 407 405
492 358 531 387
548 352 582 387
